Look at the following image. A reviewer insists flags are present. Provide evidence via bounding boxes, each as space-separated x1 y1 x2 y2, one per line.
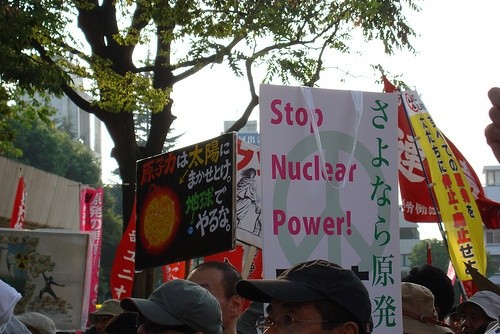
383 75 500 298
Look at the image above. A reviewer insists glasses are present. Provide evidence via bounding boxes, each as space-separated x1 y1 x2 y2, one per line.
255 313 352 333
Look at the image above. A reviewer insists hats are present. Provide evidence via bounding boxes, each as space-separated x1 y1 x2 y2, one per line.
120 279 223 334
90 299 125 315
453 290 500 321
401 282 457 334
13 312 56 334
234 259 371 322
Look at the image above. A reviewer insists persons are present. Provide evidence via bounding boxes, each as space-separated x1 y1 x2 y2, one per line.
401 265 455 322
1 279 30 333
401 282 453 334
105 310 138 334
234 259 372 334
13 311 56 334
458 291 500 333
119 279 223 334
185 261 242 334
85 299 122 333
